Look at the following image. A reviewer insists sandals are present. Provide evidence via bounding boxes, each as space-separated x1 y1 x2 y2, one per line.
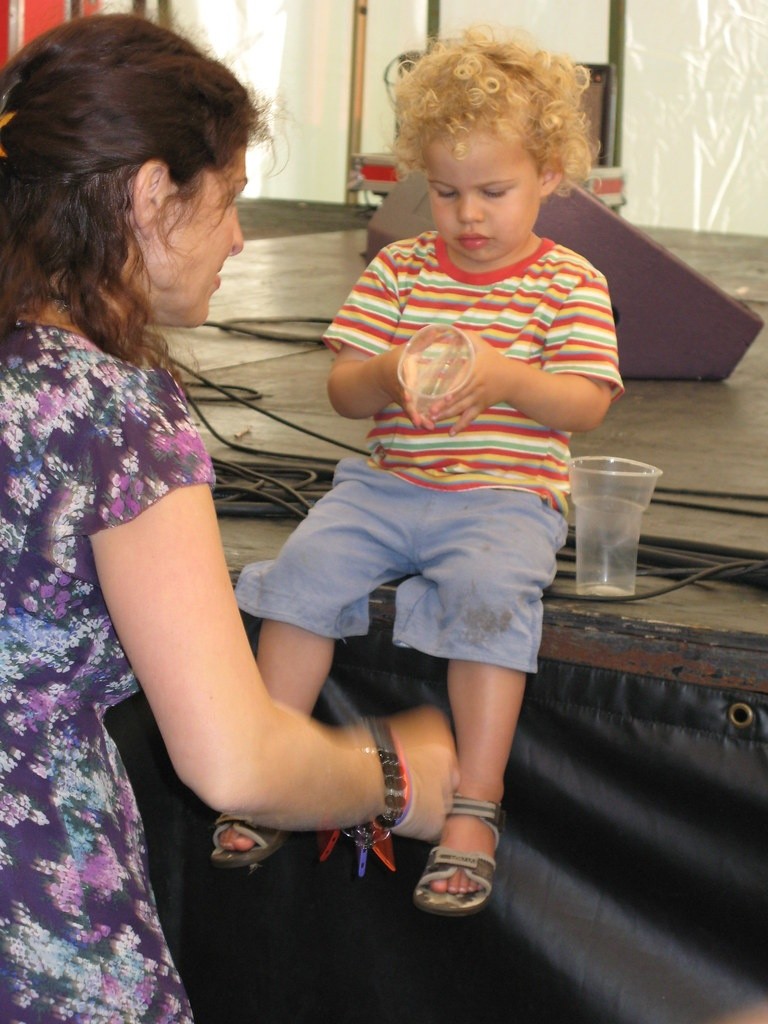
413 796 506 918
210 813 292 867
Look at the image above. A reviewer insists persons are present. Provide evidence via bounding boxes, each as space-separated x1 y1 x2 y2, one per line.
1 15 462 1024
210 35 628 915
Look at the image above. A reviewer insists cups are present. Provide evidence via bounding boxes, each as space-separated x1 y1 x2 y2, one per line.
567 455 664 597
397 324 475 418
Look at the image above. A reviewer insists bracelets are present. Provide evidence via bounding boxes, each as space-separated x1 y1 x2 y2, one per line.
341 711 412 850
